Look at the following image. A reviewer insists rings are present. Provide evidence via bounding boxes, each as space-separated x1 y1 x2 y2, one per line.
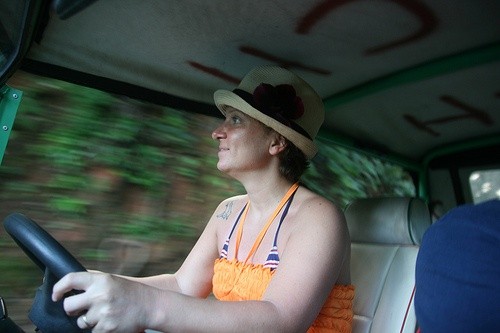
81 315 92 326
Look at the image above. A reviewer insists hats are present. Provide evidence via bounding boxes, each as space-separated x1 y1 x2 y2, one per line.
214 64 325 159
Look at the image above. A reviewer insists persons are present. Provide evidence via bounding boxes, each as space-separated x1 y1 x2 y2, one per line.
52 65 354 333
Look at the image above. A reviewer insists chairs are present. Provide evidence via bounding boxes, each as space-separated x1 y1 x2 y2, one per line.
345 195 439 333
